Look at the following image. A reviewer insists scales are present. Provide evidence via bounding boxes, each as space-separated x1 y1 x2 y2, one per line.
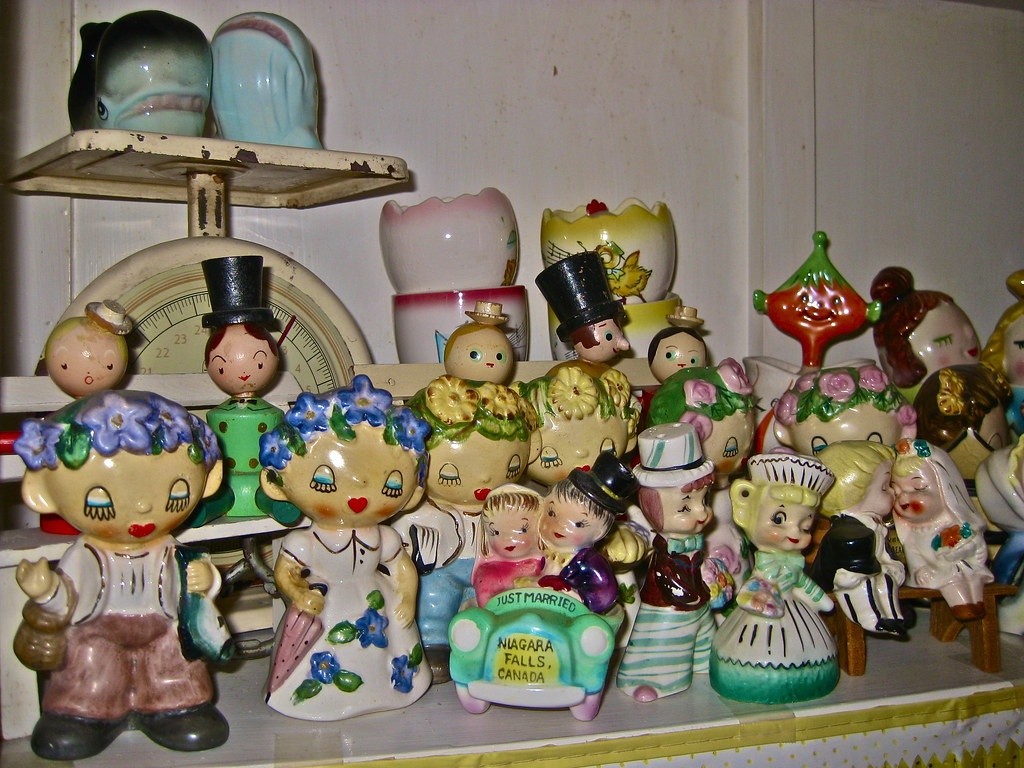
1 127 412 662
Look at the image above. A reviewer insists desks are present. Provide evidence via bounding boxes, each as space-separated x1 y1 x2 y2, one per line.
0 608 1024 768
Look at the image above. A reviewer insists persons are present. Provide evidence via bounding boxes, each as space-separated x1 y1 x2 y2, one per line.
13 253 1024 760
